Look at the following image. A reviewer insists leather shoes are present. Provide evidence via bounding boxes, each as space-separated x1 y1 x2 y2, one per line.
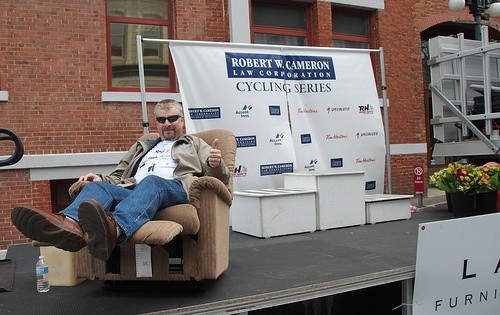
78 199 117 262
11 206 86 252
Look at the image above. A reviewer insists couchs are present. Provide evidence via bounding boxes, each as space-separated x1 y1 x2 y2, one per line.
31 128 238 287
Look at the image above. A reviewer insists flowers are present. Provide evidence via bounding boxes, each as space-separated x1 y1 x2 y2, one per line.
428 161 500 198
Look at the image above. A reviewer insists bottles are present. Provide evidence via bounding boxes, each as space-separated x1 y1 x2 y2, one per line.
35 256 50 292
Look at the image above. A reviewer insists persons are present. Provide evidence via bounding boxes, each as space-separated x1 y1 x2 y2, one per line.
10 99 230 261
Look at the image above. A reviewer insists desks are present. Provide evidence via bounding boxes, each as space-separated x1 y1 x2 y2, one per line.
365 193 415 225
232 188 316 239
282 170 367 231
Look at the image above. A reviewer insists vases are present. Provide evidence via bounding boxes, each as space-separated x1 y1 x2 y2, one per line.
452 195 474 219
446 191 453 211
476 192 497 215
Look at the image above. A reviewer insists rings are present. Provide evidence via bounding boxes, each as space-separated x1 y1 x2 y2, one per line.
212 158 215 162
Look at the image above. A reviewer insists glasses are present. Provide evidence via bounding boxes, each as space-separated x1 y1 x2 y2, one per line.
156 115 181 123
